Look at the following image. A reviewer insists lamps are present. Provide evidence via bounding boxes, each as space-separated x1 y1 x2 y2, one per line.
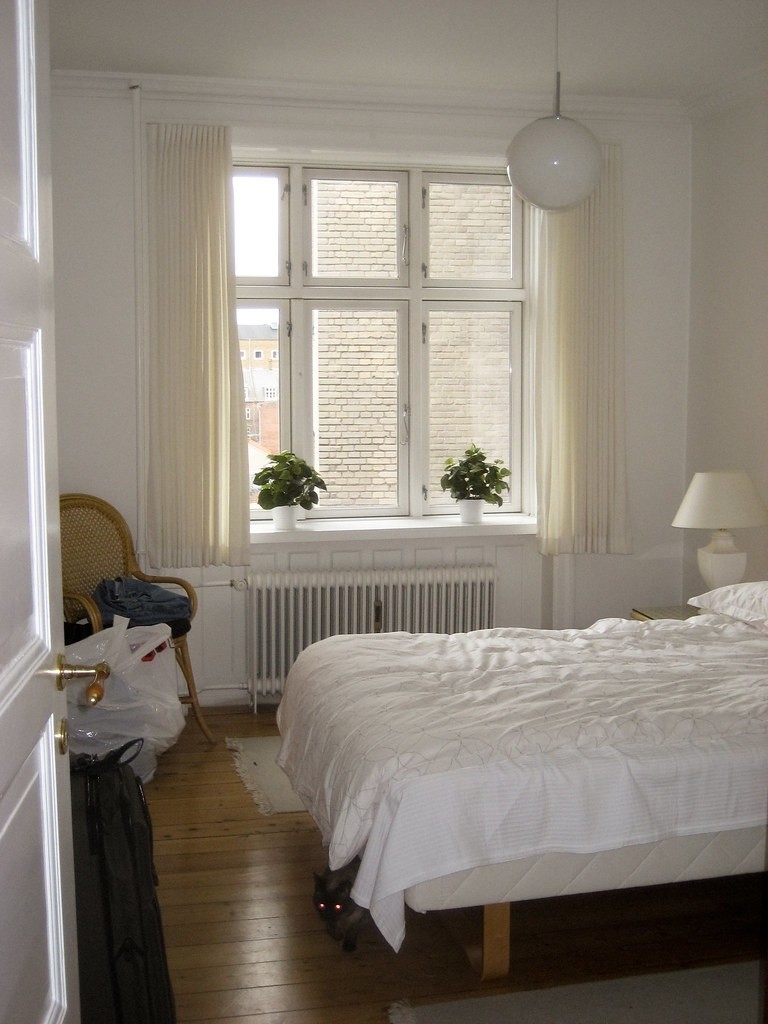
671 467 768 591
505 0 604 214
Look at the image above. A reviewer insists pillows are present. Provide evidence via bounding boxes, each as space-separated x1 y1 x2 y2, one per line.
687 581 768 637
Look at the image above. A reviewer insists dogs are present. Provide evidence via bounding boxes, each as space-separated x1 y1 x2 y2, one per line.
313 859 379 950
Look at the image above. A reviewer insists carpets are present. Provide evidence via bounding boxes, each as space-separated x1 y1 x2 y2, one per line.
224 734 311 815
387 955 768 1024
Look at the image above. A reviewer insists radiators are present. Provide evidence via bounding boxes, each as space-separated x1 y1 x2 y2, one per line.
231 564 499 716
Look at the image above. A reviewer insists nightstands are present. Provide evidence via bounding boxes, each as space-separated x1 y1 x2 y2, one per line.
630 604 718 625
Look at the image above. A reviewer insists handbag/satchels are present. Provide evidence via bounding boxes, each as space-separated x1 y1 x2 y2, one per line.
63 614 186 784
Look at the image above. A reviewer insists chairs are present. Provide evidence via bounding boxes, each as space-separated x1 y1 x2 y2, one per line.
59 492 221 746
278 579 768 982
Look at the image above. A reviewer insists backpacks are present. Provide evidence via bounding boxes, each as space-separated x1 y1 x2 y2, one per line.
68 738 176 1024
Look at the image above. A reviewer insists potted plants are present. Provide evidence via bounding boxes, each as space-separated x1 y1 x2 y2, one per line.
253 448 327 532
439 439 512 524
